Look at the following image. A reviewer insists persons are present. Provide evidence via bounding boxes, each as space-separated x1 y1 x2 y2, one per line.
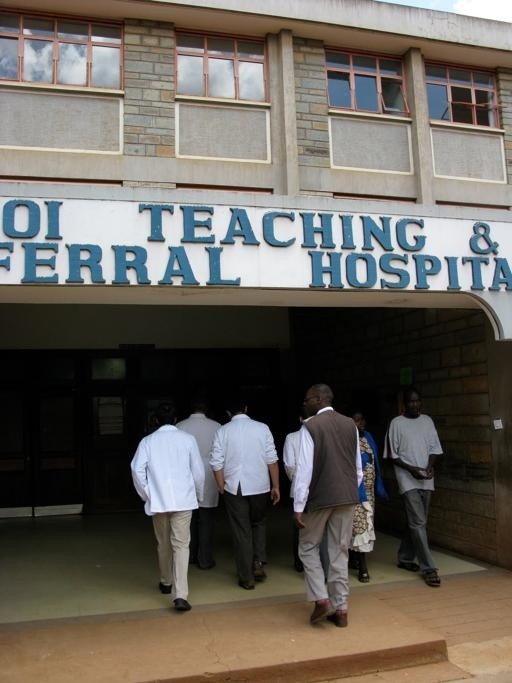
282 407 330 582
293 384 364 628
131 402 205 612
209 398 280 590
174 396 222 570
349 412 387 584
384 388 444 587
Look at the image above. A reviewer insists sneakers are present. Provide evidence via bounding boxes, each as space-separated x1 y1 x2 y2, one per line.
397 561 419 572
160 583 172 593
327 614 347 627
174 598 191 611
423 570 440 586
310 600 333 624
358 568 369 582
